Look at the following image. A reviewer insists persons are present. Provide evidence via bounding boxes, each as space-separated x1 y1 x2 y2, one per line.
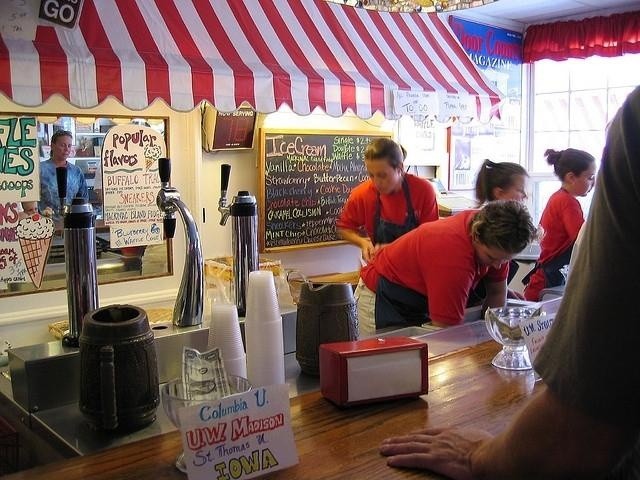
475 159 529 302
335 137 439 266
523 148 596 303
376 82 636 480
35 130 89 213
354 200 537 338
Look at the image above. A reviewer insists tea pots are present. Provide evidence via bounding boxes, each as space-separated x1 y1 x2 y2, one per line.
78 303 164 441
296 266 363 381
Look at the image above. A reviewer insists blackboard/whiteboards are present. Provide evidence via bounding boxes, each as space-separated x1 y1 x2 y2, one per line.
259 128 395 252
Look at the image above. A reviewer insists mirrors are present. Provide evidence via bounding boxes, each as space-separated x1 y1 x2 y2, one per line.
0 112 176 297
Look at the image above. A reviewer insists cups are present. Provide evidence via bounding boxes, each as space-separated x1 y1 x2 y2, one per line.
245 271 285 391
483 307 551 373
206 303 249 384
161 372 254 472
